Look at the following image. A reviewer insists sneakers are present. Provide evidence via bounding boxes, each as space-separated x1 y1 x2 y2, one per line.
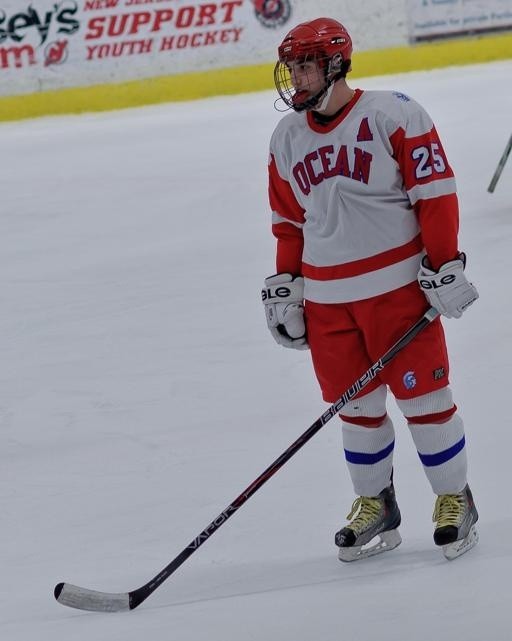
432 483 478 546
335 484 401 547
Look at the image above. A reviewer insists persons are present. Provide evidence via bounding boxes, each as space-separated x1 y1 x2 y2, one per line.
257 17 482 546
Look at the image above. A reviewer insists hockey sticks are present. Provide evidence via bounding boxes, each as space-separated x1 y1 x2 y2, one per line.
53 309 442 612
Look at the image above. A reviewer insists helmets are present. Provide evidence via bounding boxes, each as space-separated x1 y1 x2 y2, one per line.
278 17 353 113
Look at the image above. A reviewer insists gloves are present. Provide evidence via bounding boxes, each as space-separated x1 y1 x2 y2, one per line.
261 271 310 351
416 252 479 320
